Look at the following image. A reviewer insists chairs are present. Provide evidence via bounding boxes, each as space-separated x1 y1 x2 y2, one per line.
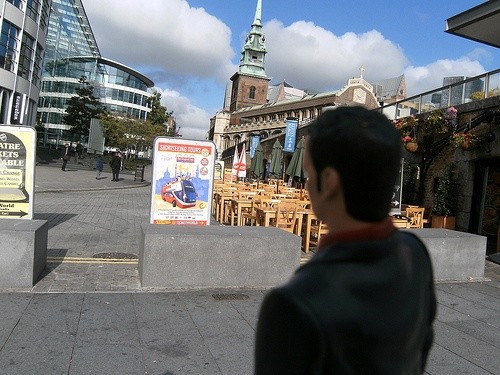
212 176 431 253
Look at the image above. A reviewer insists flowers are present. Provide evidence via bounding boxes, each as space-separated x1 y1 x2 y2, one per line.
401 135 416 144
426 106 458 134
393 114 419 130
449 130 482 147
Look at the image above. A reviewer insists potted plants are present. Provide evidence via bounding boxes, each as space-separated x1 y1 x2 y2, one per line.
427 163 457 231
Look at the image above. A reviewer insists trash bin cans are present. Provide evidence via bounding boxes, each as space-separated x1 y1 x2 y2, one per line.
134 165 145 182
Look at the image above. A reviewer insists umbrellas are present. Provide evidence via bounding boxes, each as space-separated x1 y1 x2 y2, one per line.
269 138 284 179
285 136 309 199
251 142 264 189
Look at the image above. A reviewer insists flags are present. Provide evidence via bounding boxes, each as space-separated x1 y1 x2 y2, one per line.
282 120 298 152
237 143 247 178
250 134 261 158
232 145 240 177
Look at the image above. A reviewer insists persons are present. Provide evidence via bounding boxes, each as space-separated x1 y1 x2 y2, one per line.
253 106 438 375
108 153 121 182
62 144 71 171
96 155 104 180
74 140 82 159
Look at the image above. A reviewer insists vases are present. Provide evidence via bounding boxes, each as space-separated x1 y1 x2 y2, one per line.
461 143 475 150
402 125 415 138
405 142 419 152
